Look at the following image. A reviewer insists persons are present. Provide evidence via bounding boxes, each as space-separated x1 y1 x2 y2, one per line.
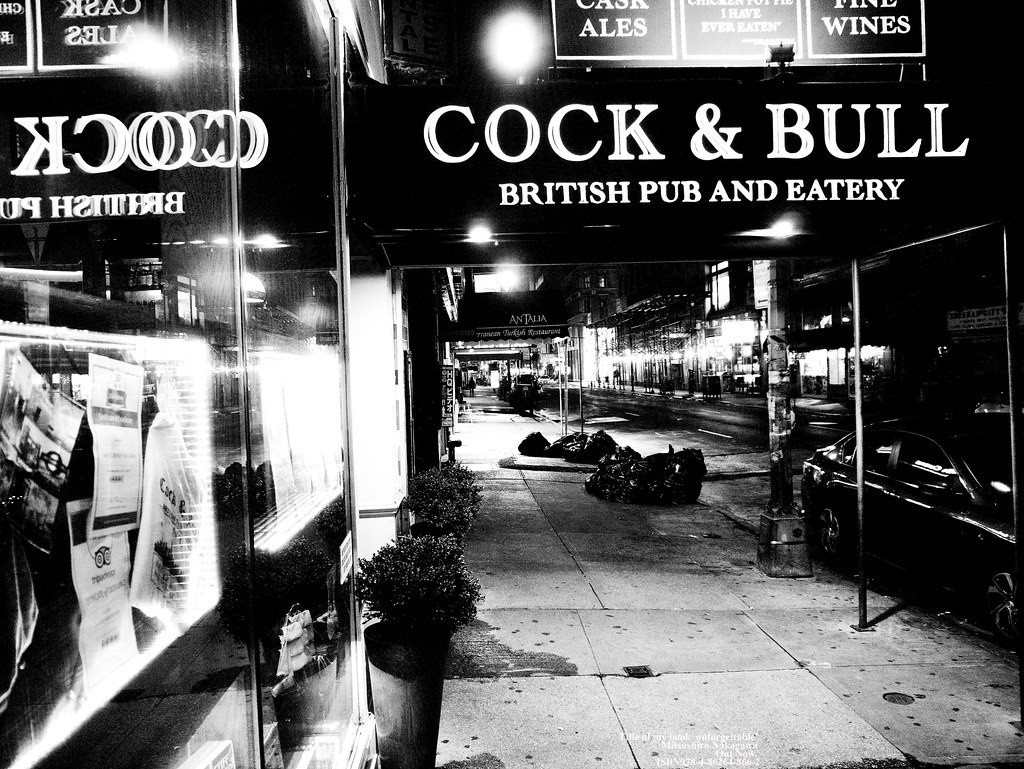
468 377 475 397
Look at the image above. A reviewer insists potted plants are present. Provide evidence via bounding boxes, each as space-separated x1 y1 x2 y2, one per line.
402 460 484 545
353 534 485 769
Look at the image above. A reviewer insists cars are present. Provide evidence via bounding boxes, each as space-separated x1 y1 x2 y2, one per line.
800 416 1024 644
